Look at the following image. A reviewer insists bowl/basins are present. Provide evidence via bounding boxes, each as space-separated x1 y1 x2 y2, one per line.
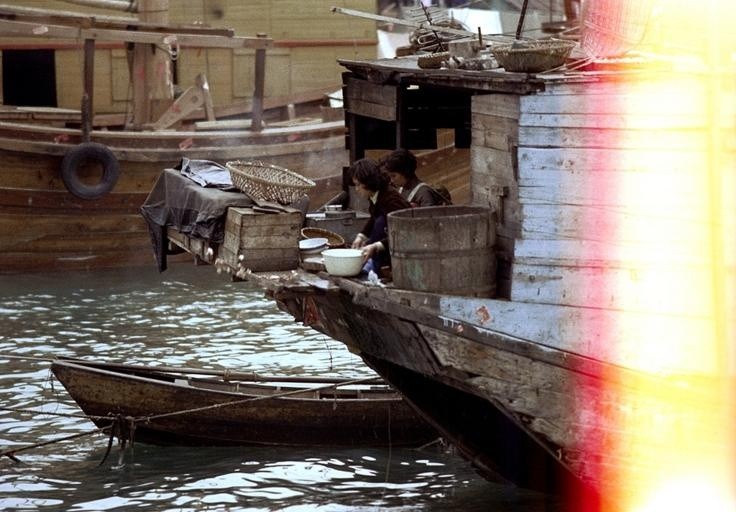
321 248 369 278
324 205 342 211
297 237 331 254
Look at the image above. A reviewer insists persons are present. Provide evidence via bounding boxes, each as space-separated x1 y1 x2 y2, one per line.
348 155 411 285
386 149 444 207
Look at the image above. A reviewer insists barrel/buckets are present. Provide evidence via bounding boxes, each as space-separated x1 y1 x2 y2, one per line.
385 204 499 299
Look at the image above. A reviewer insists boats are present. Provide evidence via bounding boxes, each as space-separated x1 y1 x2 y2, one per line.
141 0 735 510
0 0 379 122
45 354 452 454
0 0 354 275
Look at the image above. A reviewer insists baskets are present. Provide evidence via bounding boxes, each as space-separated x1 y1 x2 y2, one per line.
226 162 317 204
491 40 576 73
300 228 345 249
